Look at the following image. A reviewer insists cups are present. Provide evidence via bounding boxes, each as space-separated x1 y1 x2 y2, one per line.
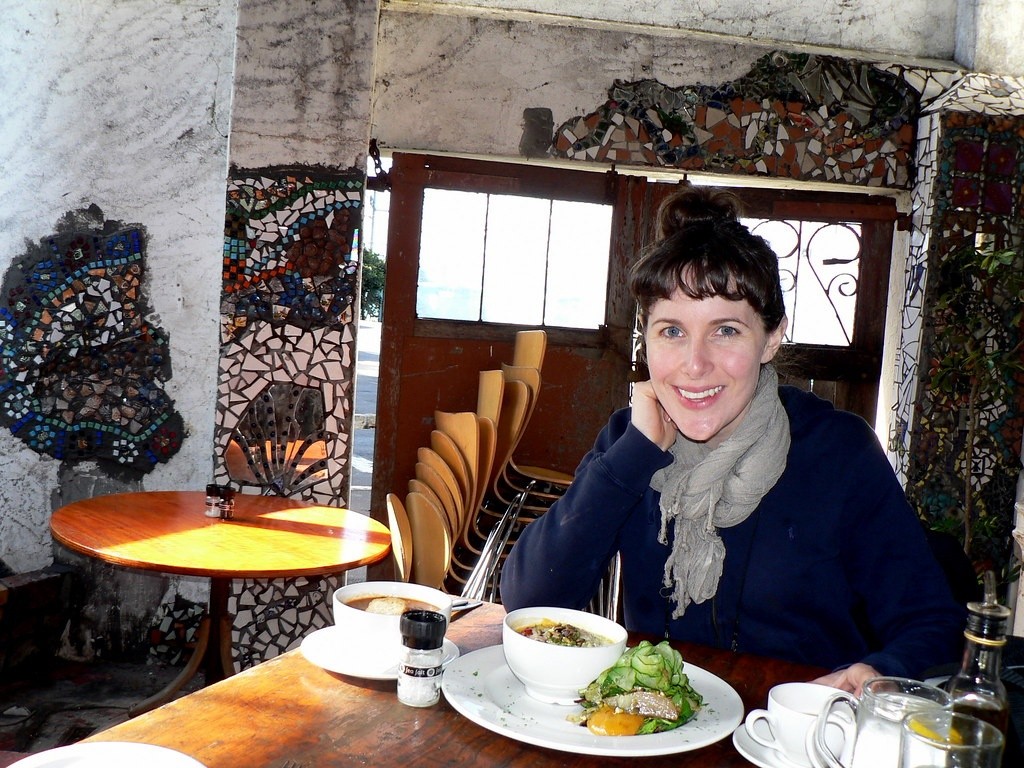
746 683 854 767
816 676 955 768
899 710 1005 768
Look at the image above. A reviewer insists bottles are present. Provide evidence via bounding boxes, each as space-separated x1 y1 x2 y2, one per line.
219 488 236 519
943 577 1010 735
396 610 446 708
204 484 220 517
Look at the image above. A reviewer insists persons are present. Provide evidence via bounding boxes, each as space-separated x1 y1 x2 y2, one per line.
496 181 968 701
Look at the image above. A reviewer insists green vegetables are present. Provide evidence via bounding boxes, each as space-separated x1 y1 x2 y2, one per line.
570 674 703 733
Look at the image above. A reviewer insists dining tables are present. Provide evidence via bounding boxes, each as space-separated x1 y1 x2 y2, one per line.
73 594 1024 768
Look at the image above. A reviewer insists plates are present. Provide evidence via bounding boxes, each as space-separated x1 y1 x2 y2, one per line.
301 626 459 681
733 719 804 768
441 644 744 757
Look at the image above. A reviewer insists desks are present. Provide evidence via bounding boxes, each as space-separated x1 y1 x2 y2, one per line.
48 491 392 737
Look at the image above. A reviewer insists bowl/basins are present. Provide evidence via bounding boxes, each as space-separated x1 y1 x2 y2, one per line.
504 607 628 705
333 582 452 672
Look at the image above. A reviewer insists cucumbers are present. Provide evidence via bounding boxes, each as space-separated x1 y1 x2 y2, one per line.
608 641 690 691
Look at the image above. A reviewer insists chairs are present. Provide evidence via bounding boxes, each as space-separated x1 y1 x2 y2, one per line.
386 493 412 583
405 329 574 605
